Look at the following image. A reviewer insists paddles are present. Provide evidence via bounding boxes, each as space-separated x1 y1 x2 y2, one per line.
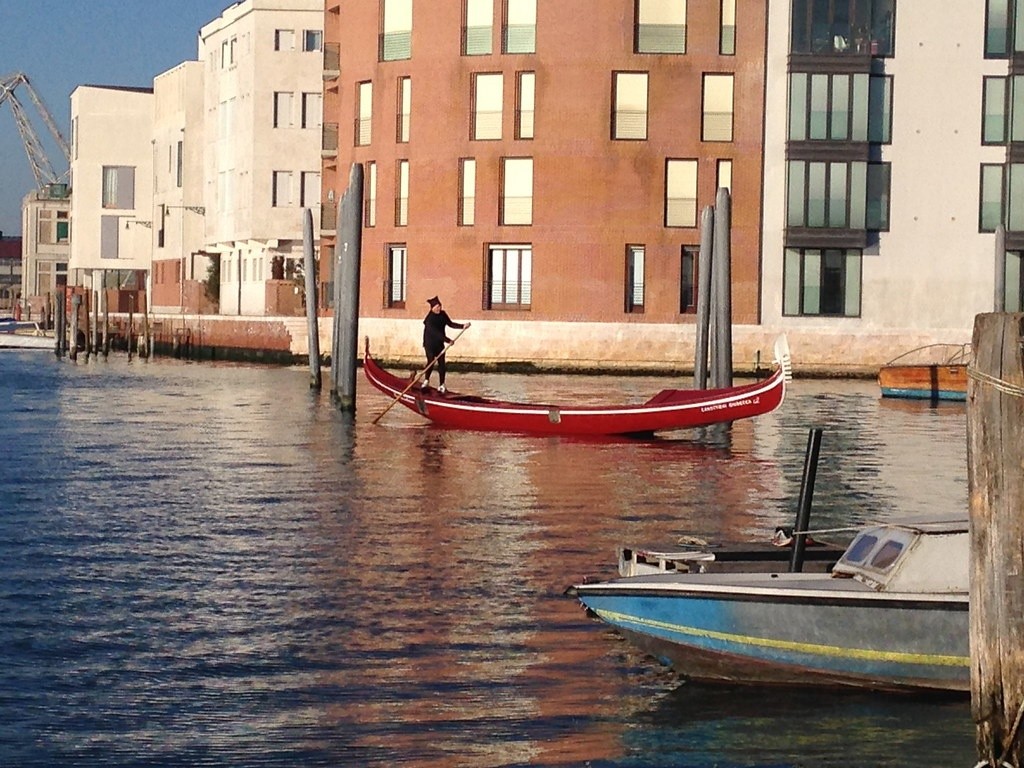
371 322 472 424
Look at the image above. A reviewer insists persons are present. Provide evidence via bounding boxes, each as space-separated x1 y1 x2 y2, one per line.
422 294 471 399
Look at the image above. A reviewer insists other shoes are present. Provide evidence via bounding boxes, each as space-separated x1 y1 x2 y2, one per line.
421 380 429 389
437 383 446 395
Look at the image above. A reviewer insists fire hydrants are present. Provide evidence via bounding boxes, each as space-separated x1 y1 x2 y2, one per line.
16 304 22 321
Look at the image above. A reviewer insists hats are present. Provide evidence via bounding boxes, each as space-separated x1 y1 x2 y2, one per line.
427 296 441 308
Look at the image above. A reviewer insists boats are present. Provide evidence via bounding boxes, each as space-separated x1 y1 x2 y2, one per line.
616 522 849 578
362 334 793 437
877 362 967 402
0 320 81 349
563 521 971 698
